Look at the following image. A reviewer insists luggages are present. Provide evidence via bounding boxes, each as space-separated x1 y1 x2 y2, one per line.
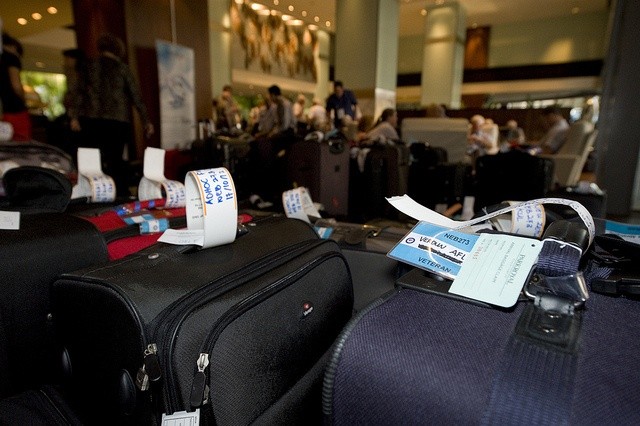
73 198 254 262
289 131 349 216
324 212 639 424
0 385 84 423
350 140 399 223
49 210 355 425
0 211 110 397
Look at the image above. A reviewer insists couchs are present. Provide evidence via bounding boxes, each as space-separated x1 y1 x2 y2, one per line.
468 123 498 147
535 120 599 190
400 118 472 164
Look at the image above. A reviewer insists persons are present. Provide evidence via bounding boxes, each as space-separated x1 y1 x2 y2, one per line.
292 93 306 120
530 103 570 155
467 112 499 175
249 99 268 121
267 84 298 139
307 96 335 131
325 80 358 128
369 108 401 140
502 119 526 152
223 86 241 128
69 34 155 195
0 34 31 142
213 100 219 121
217 91 236 132
